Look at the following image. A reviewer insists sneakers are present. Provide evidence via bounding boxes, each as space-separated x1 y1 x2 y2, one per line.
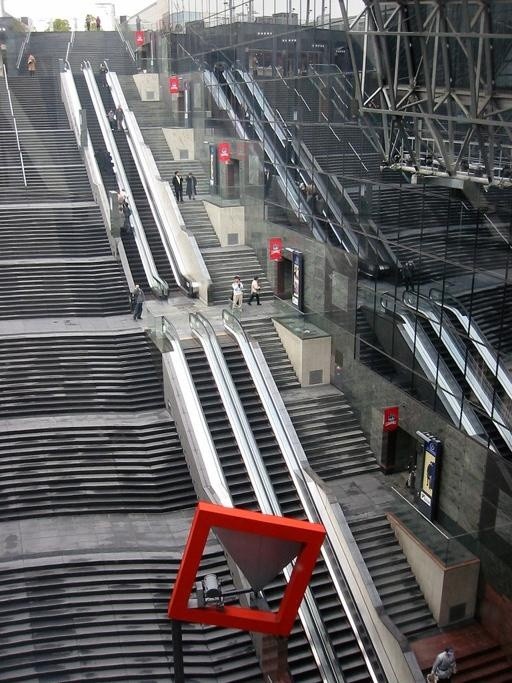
133 317 143 320
247 302 252 305
256 303 262 306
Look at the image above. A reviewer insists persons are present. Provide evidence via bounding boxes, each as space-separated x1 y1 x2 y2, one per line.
185 172 197 201
97 63 109 88
402 257 416 292
120 189 126 200
86 15 91 30
247 275 262 305
428 646 457 683
406 465 417 488
108 111 115 130
299 181 318 208
115 105 124 113
133 285 145 319
96 16 101 31
122 203 132 231
232 275 244 308
118 193 123 210
405 454 415 485
116 109 123 129
28 54 37 76
172 171 184 202
